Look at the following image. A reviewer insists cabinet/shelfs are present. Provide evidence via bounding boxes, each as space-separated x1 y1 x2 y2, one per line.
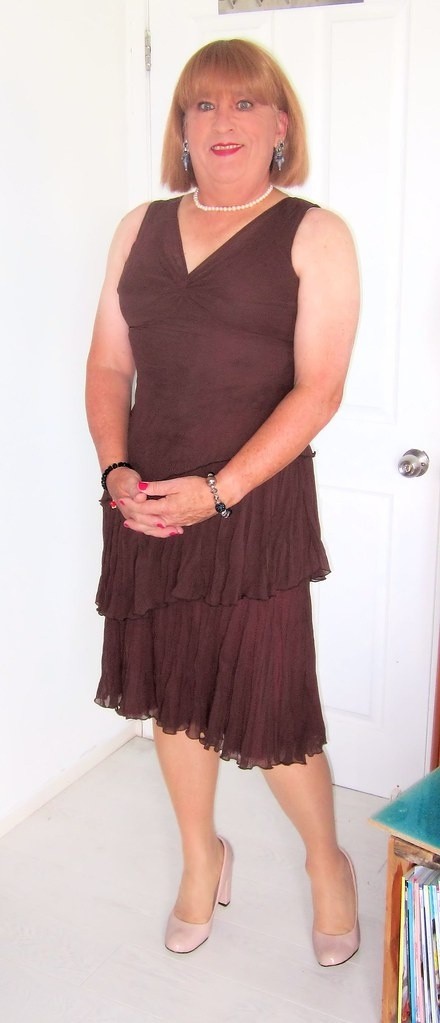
370 766 440 1023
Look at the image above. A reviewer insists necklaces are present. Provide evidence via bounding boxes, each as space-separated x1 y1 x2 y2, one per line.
194 185 274 211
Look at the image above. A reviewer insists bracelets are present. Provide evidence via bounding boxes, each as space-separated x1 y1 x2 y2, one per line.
205 472 232 518
101 463 132 491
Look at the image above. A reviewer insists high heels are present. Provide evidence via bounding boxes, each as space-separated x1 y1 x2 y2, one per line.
312 844 360 968
164 834 233 954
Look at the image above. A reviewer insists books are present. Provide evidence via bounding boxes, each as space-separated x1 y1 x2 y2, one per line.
394 865 440 1023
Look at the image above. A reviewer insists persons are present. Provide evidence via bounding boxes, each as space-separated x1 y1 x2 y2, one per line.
85 40 358 967
